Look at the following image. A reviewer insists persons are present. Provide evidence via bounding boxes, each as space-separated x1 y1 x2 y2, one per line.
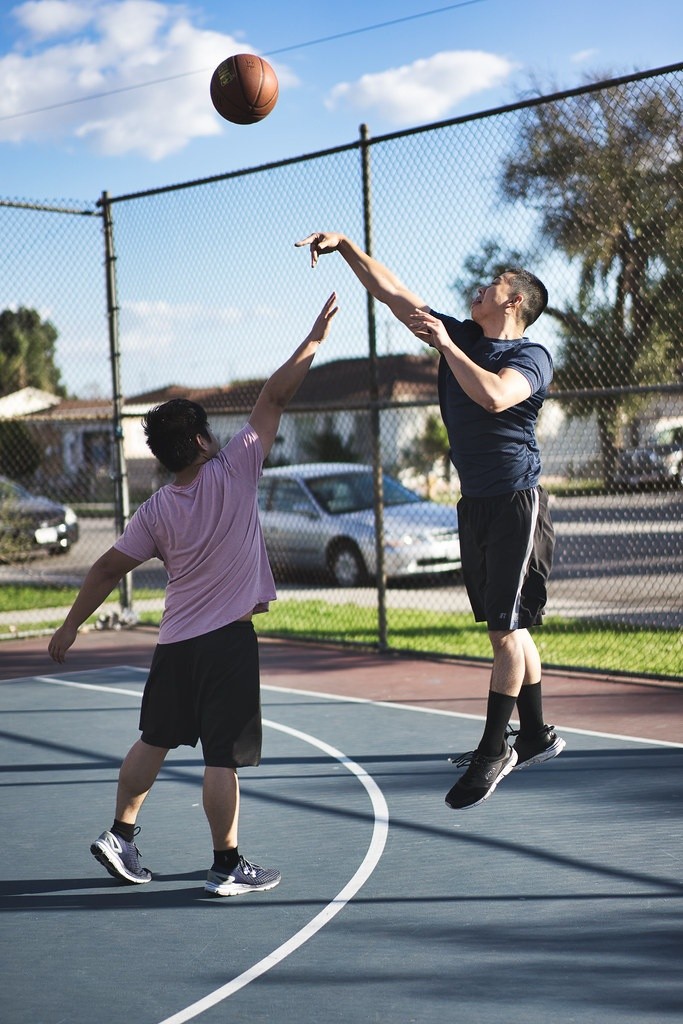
47 291 338 895
295 232 566 810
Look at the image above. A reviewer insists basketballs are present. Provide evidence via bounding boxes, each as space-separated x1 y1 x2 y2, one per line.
209 53 281 127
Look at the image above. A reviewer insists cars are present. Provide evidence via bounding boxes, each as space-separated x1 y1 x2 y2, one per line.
256 462 462 588
0 477 81 567
612 424 683 496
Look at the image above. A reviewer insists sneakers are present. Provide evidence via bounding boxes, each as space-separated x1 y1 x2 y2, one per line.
204 855 282 896
507 723 567 771
90 825 153 883
445 743 519 810
